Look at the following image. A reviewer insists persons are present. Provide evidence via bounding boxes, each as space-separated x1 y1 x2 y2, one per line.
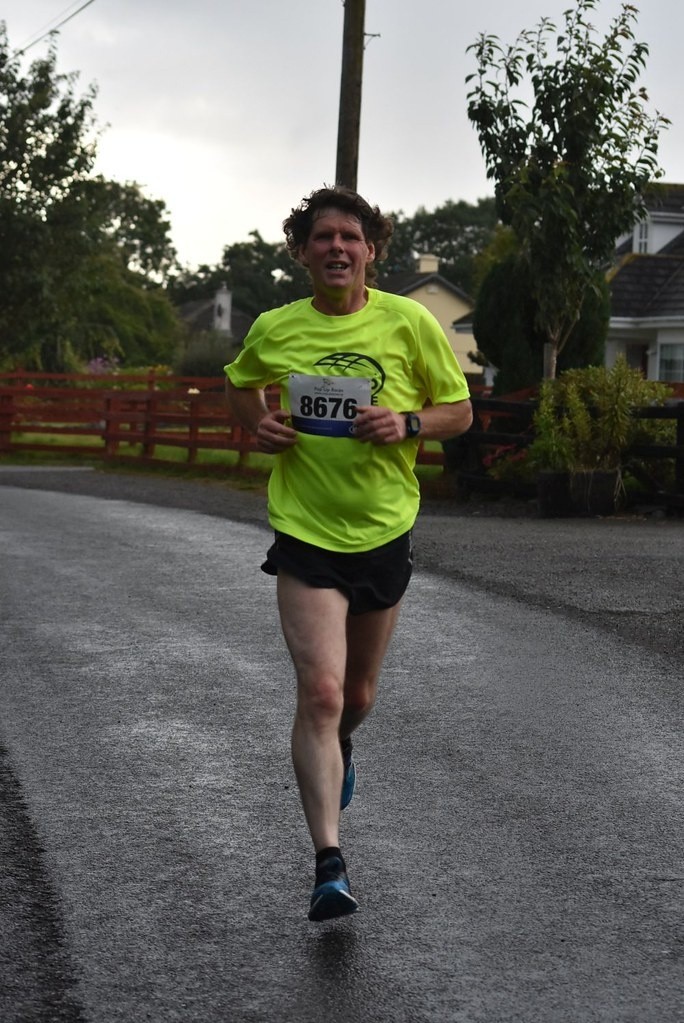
223 184 474 922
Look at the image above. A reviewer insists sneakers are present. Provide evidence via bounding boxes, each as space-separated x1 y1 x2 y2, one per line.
339 746 354 810
308 846 360 921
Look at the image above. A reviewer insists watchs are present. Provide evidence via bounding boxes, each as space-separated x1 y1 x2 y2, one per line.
399 411 422 440
